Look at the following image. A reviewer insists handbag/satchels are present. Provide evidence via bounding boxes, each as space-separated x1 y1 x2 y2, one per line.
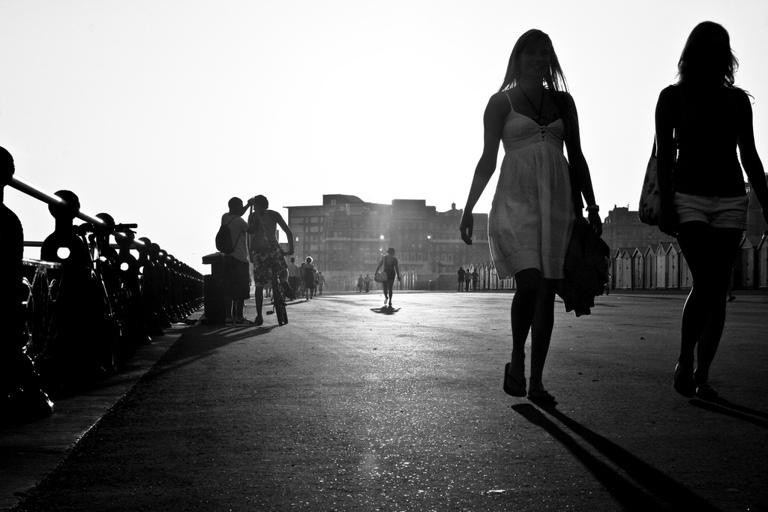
374 272 388 283
555 213 612 316
637 83 680 226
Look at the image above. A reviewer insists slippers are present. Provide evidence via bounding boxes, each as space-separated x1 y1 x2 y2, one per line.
503 362 526 398
528 389 555 403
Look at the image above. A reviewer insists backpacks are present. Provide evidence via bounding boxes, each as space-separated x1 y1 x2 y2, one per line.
215 212 244 255
254 210 278 254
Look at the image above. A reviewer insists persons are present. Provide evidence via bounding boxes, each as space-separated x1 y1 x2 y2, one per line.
218 194 402 329
457 267 478 292
652 20 768 398
460 34 604 406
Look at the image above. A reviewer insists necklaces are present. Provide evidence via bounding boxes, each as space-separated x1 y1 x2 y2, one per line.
519 84 545 123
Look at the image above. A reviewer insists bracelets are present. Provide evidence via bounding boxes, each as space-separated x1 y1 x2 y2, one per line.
585 206 600 212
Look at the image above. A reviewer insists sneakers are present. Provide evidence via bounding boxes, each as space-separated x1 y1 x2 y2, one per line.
225 282 294 327
673 360 721 403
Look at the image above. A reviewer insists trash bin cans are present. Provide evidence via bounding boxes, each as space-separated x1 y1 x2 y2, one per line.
201 252 225 325
